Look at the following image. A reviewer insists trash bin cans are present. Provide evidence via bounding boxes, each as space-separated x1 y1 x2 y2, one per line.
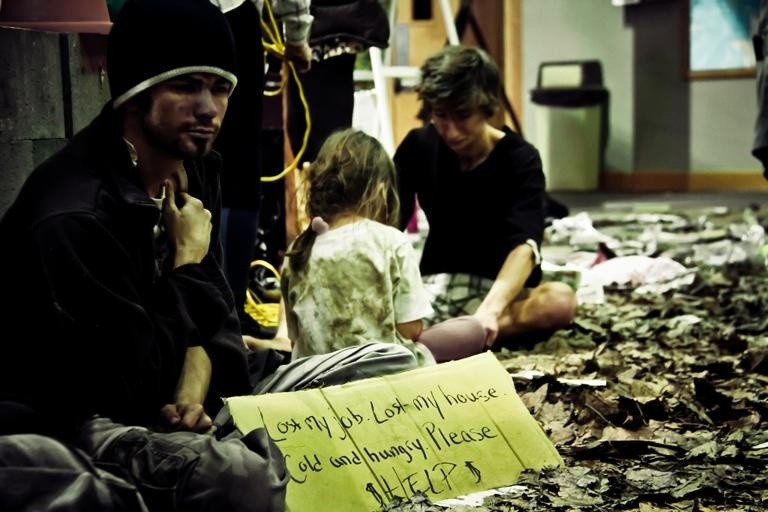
528 60 609 191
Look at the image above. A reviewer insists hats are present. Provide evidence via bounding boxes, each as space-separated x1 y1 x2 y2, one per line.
108 0 239 109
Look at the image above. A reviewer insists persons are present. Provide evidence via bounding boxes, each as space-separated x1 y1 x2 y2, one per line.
108 2 389 302
280 129 486 367
391 45 578 346
2 1 417 510
